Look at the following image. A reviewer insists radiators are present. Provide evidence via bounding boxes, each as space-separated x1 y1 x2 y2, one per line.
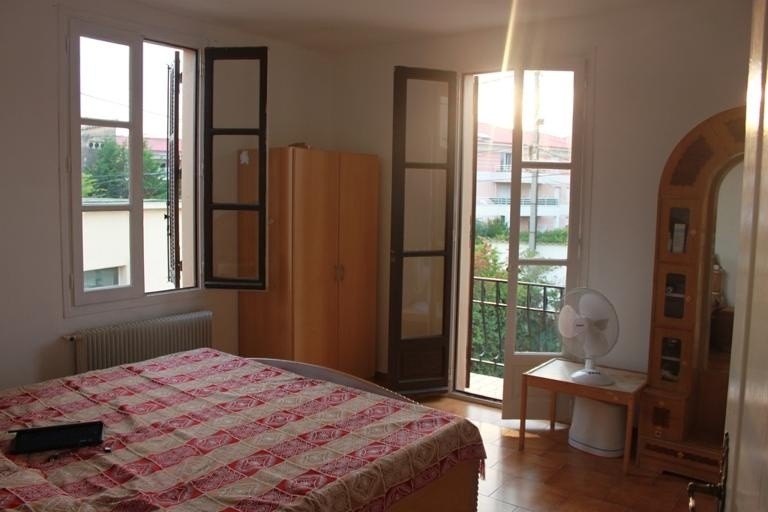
61 311 215 374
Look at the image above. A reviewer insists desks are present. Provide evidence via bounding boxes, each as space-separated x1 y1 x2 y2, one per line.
520 358 648 474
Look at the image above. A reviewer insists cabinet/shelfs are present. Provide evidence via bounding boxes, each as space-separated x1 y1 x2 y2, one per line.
238 146 380 382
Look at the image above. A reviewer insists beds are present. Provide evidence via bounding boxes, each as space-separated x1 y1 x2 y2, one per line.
1 348 486 511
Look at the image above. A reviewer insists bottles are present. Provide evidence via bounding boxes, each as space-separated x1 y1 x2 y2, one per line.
712 265 721 295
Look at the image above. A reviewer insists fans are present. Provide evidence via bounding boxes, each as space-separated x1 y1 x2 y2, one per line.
552 288 619 388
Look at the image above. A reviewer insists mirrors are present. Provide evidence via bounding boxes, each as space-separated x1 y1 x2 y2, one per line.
695 154 743 445
